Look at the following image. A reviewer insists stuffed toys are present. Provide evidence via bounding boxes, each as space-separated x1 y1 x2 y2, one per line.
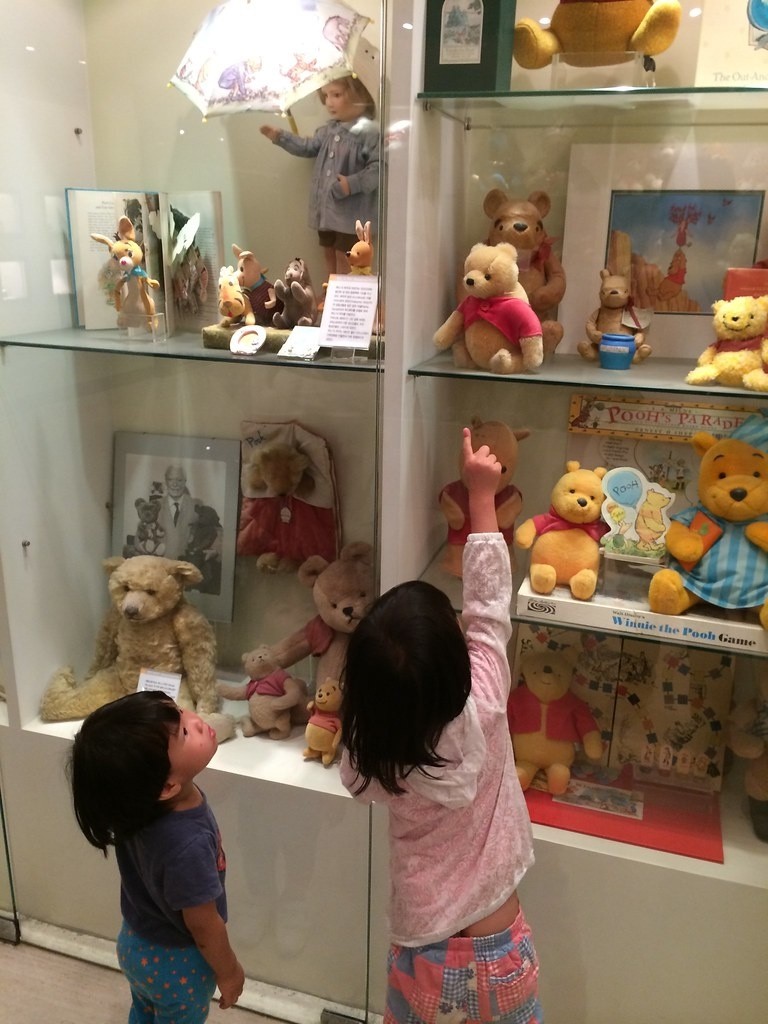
515 460 608 600
439 414 528 581
218 219 374 325
215 643 302 740
725 659 768 845
88 218 161 326
507 645 602 794
514 0 682 69
685 294 768 392
150 480 167 498
577 270 654 364
188 504 220 554
648 413 768 629
302 677 343 765
42 554 236 745
133 498 166 556
433 186 566 373
237 443 340 586
271 540 377 724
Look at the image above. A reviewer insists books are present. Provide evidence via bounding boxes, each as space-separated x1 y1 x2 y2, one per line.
64 187 224 330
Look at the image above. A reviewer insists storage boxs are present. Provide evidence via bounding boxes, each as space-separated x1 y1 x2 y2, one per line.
424 0 516 91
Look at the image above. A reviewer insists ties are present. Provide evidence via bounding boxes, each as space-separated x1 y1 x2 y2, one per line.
173 502 179 527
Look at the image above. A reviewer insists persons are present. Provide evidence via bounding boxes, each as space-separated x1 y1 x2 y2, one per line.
157 462 203 560
336 428 542 1024
260 34 379 297
65 691 245 1024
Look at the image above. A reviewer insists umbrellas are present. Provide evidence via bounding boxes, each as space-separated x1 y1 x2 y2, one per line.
169 0 374 135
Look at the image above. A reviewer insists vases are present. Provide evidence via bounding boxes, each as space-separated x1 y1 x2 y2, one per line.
599 333 636 370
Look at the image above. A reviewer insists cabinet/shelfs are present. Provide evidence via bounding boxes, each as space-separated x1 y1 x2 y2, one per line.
0 0 768 1024
